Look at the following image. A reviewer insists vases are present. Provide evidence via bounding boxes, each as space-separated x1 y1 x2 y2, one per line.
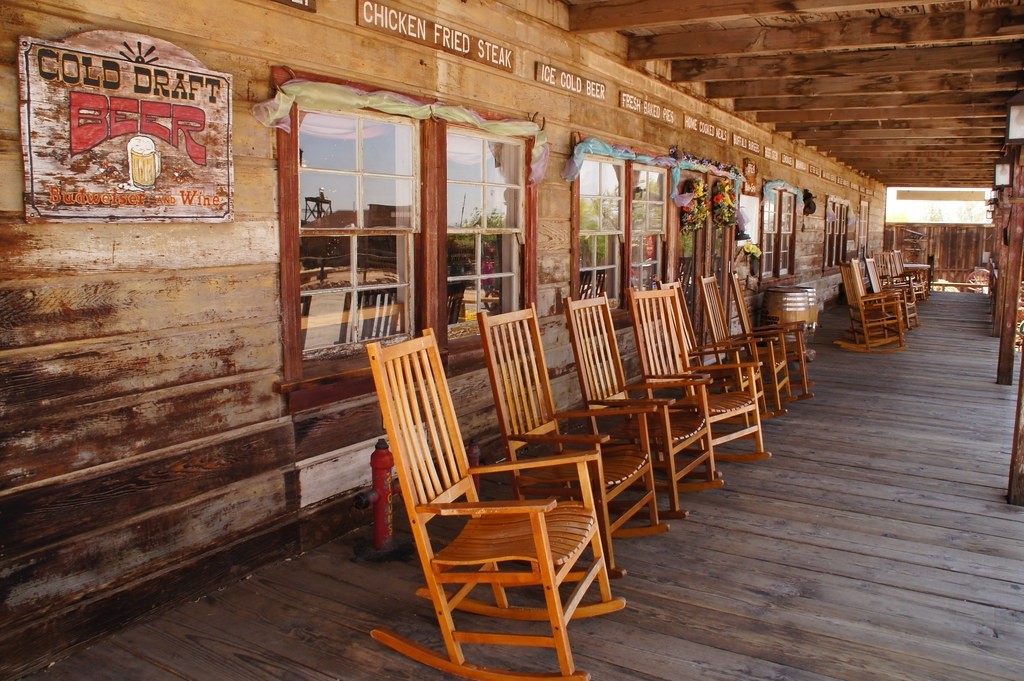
750 254 759 275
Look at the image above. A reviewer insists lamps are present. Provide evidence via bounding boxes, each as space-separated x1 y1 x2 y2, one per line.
993 156 1012 188
1006 90 1024 143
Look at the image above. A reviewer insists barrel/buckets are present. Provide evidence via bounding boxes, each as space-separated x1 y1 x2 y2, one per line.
760 286 809 347
776 285 816 337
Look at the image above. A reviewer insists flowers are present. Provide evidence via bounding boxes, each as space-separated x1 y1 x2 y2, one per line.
743 241 762 258
682 176 709 229
712 178 738 227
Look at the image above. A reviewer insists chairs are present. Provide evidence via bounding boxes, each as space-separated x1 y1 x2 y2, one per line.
483 304 671 581
564 292 724 521
364 327 626 681
832 248 928 354
624 271 815 478
295 251 605 349
